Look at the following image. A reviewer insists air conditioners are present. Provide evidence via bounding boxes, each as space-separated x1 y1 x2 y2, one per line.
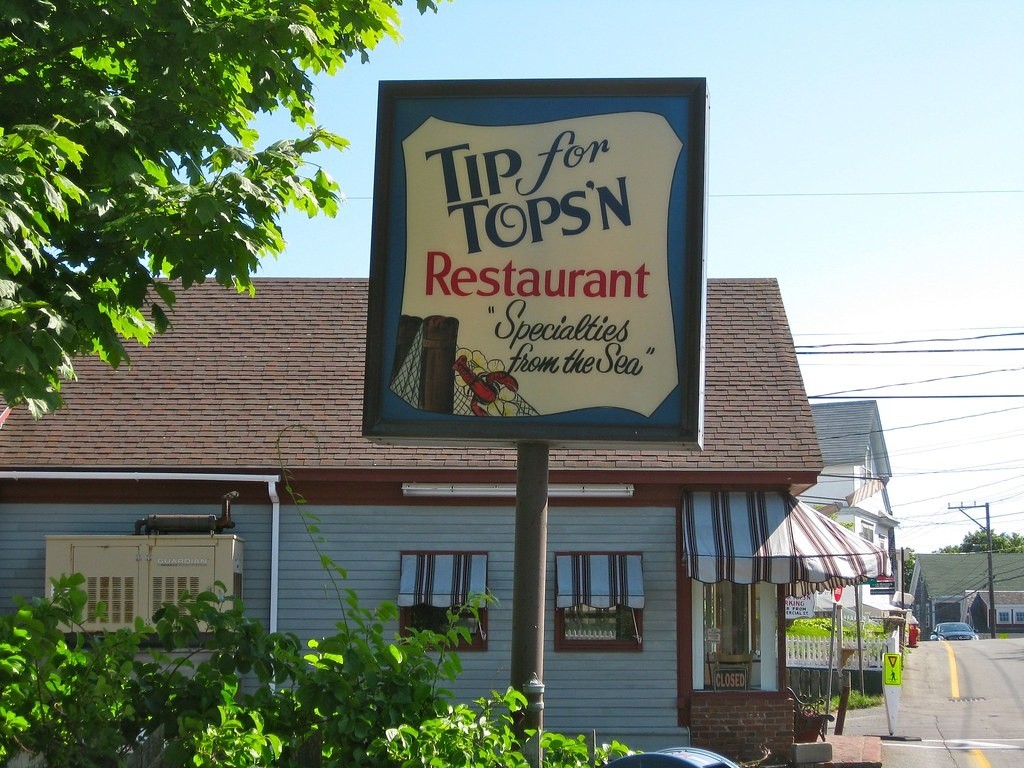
44 535 243 633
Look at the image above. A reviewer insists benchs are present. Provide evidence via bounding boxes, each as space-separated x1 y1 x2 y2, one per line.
787 687 835 742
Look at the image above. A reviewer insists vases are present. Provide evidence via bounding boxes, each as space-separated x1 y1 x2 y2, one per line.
794 712 823 742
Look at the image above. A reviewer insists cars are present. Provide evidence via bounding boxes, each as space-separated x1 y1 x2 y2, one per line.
930 621 979 641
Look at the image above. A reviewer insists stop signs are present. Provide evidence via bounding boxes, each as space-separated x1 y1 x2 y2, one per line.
834 584 843 602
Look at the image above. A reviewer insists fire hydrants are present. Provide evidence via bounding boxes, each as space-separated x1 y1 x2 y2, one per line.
905 615 921 648
